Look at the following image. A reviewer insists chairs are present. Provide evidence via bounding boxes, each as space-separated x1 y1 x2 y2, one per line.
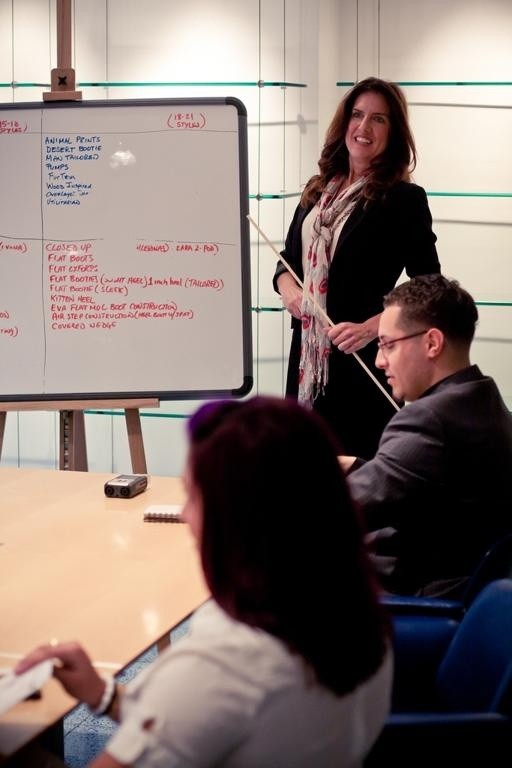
376 534 511 727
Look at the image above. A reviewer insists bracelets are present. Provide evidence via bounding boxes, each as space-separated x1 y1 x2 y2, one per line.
89 672 119 719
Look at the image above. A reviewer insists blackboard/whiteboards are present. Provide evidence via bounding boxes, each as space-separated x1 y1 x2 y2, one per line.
0 98 253 410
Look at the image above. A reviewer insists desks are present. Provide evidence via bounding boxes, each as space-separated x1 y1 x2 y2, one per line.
1 463 214 768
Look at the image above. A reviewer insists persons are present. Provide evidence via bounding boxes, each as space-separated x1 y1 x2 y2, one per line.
347 269 511 604
12 395 398 768
272 75 441 457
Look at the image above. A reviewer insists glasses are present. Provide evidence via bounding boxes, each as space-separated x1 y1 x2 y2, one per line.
377 328 429 361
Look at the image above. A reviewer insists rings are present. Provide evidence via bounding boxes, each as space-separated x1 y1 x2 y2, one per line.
49 639 59 648
